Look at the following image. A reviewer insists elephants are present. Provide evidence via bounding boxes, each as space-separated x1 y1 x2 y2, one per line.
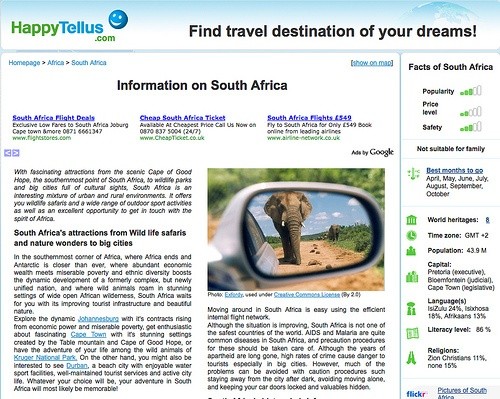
328 224 340 242
263 191 312 266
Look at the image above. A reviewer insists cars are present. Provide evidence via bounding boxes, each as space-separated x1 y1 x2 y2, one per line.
242 209 280 276
